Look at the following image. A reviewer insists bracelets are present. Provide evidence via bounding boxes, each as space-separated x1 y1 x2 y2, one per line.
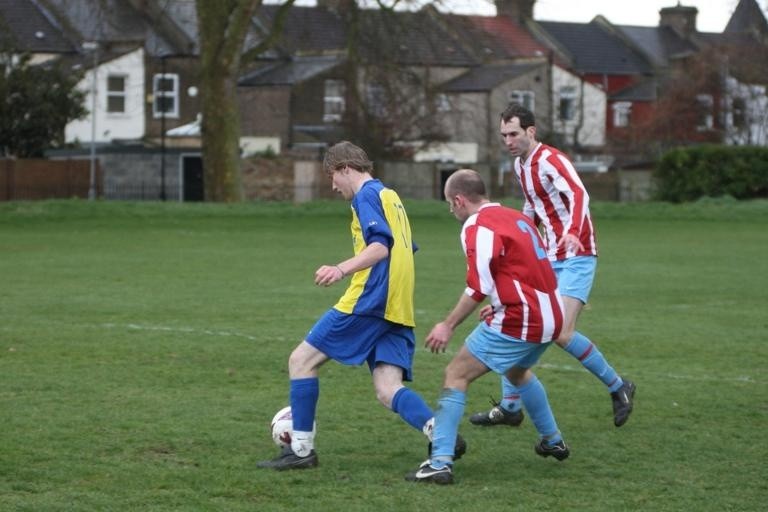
334 265 346 280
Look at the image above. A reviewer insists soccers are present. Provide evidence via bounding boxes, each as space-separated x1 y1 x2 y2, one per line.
271 405 317 450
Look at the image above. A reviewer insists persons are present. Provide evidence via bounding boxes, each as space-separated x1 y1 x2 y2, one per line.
258 141 469 469
403 168 570 483
466 102 636 425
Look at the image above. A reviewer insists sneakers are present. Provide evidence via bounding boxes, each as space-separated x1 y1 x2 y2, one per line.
256 443 320 472
427 432 468 461
533 439 571 462
609 377 637 428
468 402 525 428
402 458 456 486
